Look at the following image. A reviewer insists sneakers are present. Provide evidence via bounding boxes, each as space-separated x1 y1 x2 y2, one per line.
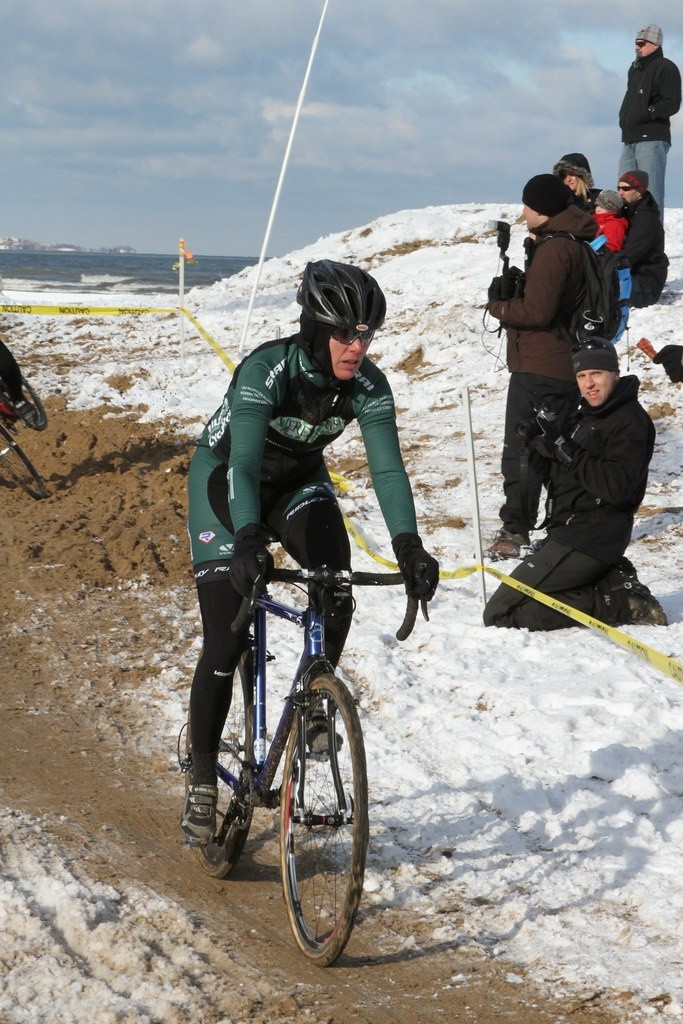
179 767 219 842
482 525 531 560
591 567 668 627
595 554 650 594
302 700 341 753
10 395 39 428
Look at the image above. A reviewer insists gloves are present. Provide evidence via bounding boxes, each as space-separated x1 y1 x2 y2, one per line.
487 276 501 301
228 523 275 597
392 532 440 602
526 416 588 468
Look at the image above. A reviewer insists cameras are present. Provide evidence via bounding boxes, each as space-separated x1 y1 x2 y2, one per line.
486 219 526 301
514 404 558 440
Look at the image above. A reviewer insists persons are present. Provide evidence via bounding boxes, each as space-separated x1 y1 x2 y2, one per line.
591 190 628 252
179 259 440 846
553 153 603 212
619 24 682 225
484 335 668 632
0 340 38 428
482 174 600 557
618 170 670 308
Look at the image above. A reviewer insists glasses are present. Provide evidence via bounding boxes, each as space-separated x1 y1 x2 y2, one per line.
635 40 654 48
617 185 634 192
569 340 616 356
316 320 376 348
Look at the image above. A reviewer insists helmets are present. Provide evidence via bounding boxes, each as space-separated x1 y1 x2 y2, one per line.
296 260 386 331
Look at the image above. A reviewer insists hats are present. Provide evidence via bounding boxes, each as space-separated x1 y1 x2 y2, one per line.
619 170 648 195
636 23 663 47
522 173 574 217
595 189 623 212
571 337 620 374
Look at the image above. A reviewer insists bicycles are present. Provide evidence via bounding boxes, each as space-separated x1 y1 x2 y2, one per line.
175 550 431 968
0 372 48 500
